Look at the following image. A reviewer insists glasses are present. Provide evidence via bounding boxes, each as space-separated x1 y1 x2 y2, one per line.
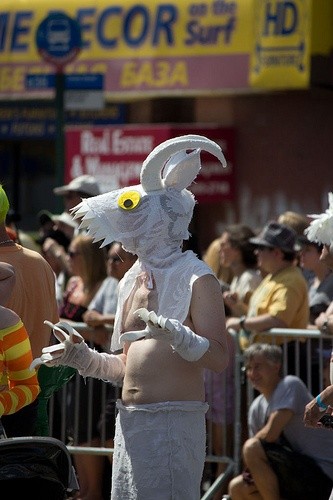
104 255 136 265
66 250 87 260
253 244 276 252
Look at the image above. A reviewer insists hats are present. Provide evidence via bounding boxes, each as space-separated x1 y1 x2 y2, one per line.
57 212 80 228
54 175 99 198
249 224 303 253
279 211 311 240
304 192 333 246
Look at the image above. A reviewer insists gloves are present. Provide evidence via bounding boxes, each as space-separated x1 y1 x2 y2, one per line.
29 319 126 383
118 307 210 362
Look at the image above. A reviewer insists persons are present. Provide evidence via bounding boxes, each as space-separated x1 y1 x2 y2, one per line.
42 133 228 500
201 192 333 500
0 305 41 440
229 343 333 500
0 174 137 500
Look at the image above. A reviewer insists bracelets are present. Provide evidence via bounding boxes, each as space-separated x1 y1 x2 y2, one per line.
240 316 246 328
322 318 329 333
0 239 13 245
315 394 328 411
